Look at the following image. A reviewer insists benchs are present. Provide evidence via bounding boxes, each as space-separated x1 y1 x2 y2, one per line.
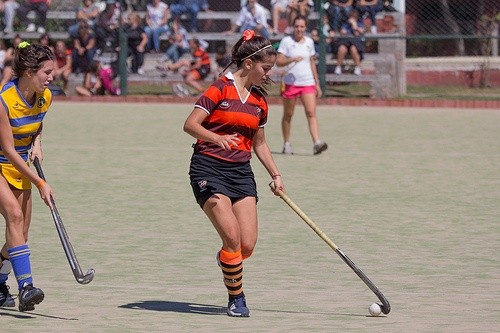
0 0 393 84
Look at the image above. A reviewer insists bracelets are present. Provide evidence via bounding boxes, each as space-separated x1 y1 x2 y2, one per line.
36 180 44 188
272 174 280 178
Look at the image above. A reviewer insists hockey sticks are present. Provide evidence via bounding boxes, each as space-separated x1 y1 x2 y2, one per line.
270 181 391 314
31 152 96 284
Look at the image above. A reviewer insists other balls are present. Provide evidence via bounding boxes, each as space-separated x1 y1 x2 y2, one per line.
369 303 381 316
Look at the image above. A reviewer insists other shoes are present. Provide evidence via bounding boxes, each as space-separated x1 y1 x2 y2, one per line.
334 65 342 75
313 141 328 155
282 143 294 156
353 66 362 75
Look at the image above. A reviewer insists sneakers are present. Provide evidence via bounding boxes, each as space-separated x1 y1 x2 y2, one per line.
227 293 250 318
0 282 15 307
17 281 46 312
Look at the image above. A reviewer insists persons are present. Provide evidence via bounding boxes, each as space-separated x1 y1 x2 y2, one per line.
0 41 56 311
0 0 499 97
183 29 284 317
277 15 328 155
